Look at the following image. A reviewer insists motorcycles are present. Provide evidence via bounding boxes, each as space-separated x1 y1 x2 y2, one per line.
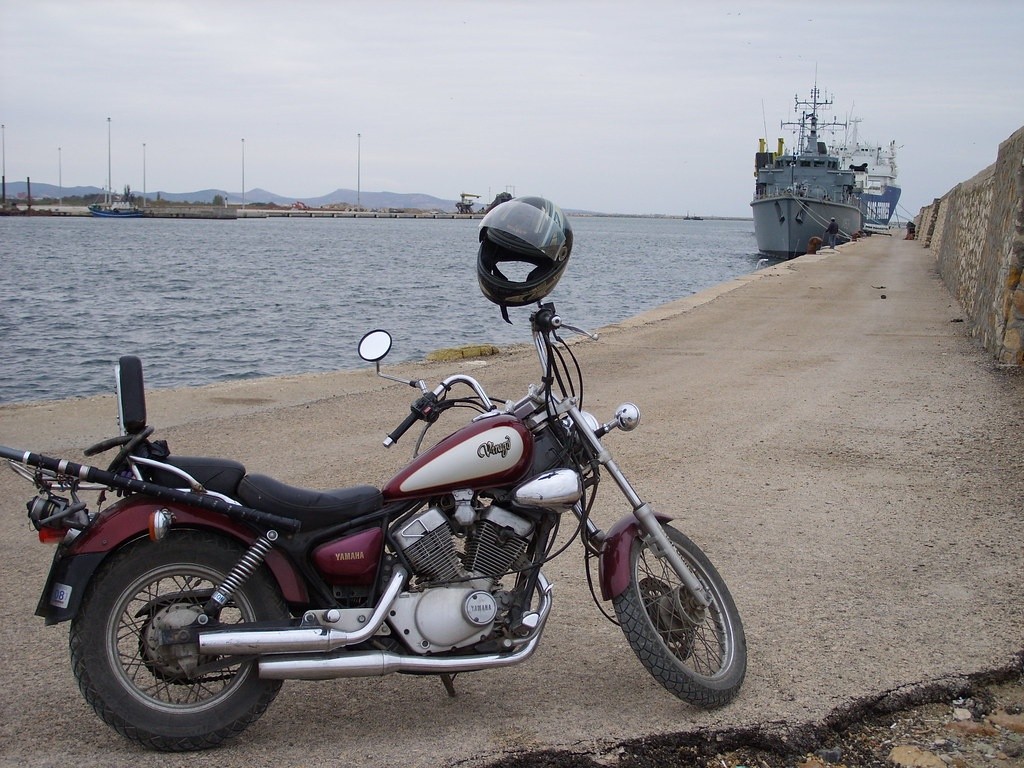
0 295 748 752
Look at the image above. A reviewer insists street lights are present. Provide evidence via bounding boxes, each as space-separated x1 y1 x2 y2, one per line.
1 125 5 175
106 117 112 204
241 137 245 210
58 148 63 205
142 143 146 207
357 133 360 211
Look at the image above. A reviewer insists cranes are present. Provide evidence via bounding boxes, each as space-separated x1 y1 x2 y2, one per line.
455 192 483 213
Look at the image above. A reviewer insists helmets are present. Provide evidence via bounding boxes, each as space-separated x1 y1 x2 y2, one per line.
476 195 574 307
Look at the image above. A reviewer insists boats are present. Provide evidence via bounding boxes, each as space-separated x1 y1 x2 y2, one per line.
750 60 902 260
88 201 144 218
683 214 704 221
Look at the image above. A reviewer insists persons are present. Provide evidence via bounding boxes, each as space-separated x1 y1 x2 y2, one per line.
826 217 838 249
